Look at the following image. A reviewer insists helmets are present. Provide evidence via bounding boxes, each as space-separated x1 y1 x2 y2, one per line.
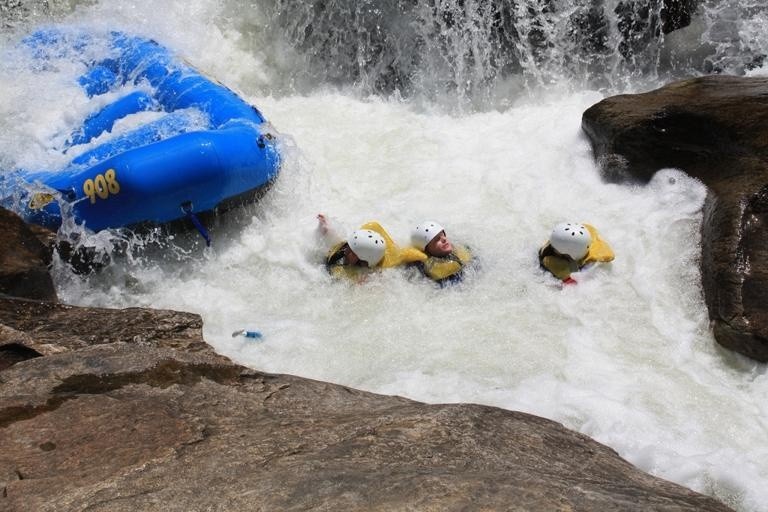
549 220 594 261
410 222 447 253
346 229 387 270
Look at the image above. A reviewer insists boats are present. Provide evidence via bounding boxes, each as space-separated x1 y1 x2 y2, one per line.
0 25 282 240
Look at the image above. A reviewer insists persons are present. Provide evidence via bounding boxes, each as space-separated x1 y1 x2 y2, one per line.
536 222 615 287
317 214 400 284
404 220 479 290
